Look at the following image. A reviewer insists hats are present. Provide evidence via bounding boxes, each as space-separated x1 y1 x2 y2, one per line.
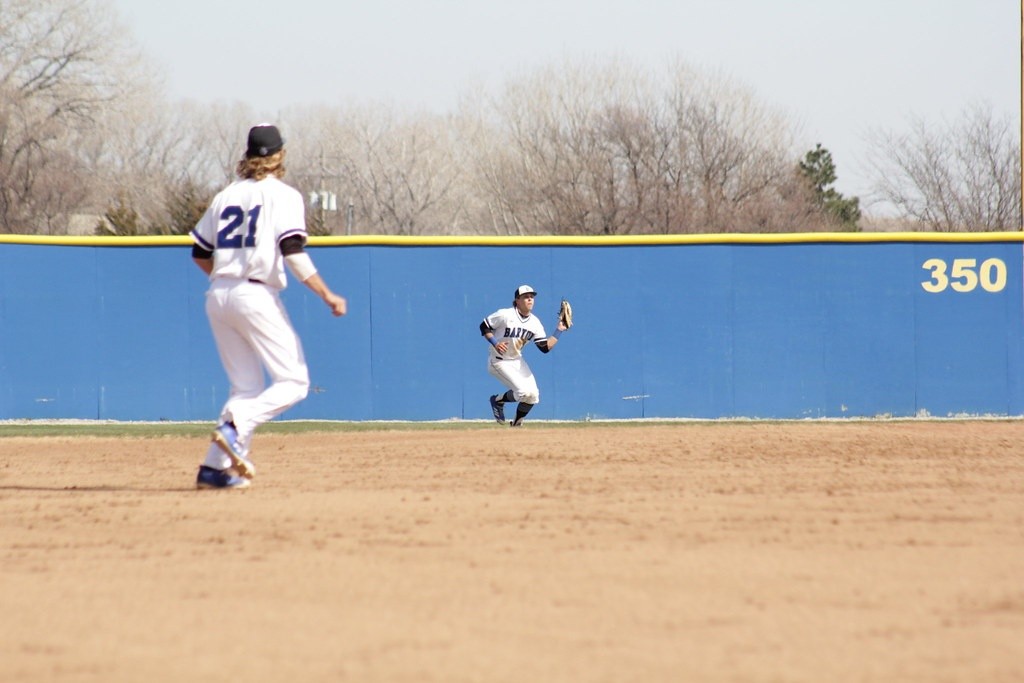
515 285 537 299
247 122 287 156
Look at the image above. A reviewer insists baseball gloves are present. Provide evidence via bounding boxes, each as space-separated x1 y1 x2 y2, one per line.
557 300 574 328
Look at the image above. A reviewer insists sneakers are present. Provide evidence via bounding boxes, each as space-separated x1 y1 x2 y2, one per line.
210 421 254 479
197 466 250 489
490 394 505 425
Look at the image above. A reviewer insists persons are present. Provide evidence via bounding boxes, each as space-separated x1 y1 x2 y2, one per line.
184 124 347 490
478 285 573 428
